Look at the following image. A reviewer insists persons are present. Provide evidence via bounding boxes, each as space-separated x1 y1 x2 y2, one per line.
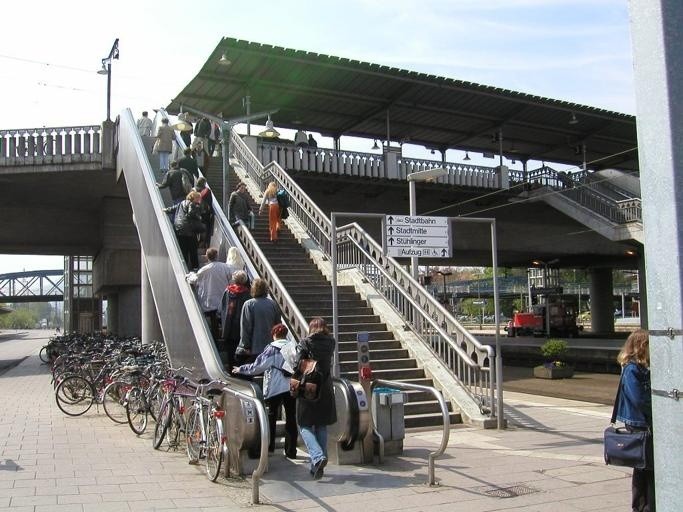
189 177 212 247
293 317 336 480
177 113 187 132
208 118 220 158
295 127 309 149
232 323 300 458
137 111 153 137
259 182 281 244
307 133 318 148
190 112 211 157
227 246 246 287
178 149 199 188
185 248 232 353
162 191 202 270
154 117 176 175
615 329 652 510
218 270 255 378
236 280 283 382
228 183 253 231
181 112 194 150
156 161 195 222
189 142 209 181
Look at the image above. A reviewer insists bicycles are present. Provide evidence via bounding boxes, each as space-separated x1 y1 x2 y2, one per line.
39 331 171 405
153 367 192 452
122 362 192 452
56 364 142 424
186 380 228 482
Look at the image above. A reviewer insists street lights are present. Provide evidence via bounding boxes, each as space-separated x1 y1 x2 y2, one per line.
98 38 122 119
405 167 444 282
174 101 278 218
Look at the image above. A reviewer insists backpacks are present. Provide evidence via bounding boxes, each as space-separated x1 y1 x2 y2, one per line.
282 346 299 377
276 190 286 214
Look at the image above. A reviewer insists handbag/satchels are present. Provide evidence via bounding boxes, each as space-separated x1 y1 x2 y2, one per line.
277 189 289 208
189 215 208 232
290 341 322 402
181 168 192 194
604 365 653 471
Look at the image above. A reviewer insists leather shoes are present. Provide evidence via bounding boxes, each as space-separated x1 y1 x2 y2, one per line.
310 468 317 475
314 456 327 481
